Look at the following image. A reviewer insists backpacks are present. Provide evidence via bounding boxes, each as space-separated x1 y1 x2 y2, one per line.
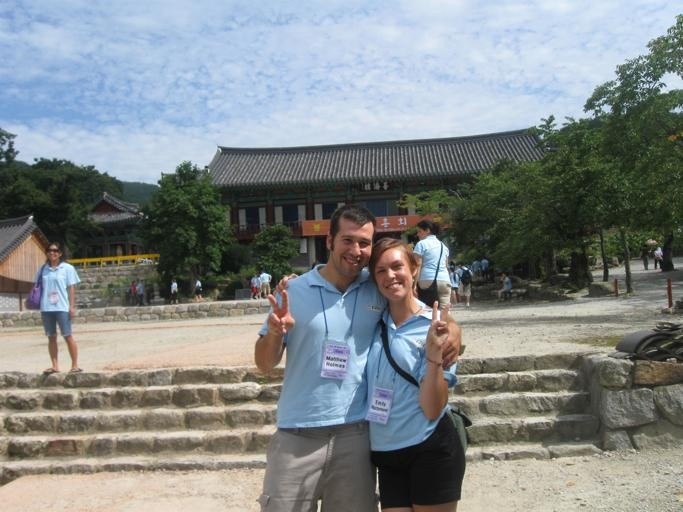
458 269 471 285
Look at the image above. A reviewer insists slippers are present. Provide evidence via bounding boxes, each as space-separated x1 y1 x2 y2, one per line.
43 368 61 374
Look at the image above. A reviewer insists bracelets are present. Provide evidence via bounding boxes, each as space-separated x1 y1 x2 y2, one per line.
427 356 446 368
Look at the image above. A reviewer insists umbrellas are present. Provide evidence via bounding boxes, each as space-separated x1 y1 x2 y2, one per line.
645 239 658 245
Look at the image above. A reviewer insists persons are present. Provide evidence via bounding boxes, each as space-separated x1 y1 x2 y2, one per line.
252 269 272 299
654 247 663 269
194 280 203 302
366 238 466 512
446 257 491 308
130 280 137 306
412 220 451 312
137 277 146 306
168 279 177 304
497 272 512 301
35 241 82 376
255 205 461 512
640 245 650 270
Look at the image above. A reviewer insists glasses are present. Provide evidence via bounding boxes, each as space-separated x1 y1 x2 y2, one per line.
47 249 59 252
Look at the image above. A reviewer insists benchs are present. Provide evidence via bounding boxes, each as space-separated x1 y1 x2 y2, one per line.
490 288 526 300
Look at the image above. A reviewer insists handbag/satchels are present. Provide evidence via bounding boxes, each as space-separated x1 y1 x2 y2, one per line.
450 408 471 453
26 286 42 309
415 279 440 309
